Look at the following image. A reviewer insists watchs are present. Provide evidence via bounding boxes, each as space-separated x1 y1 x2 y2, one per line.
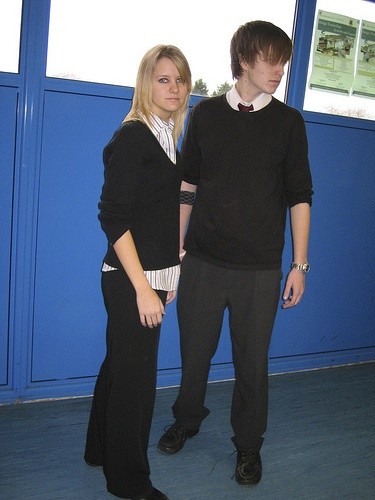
290 261 310 273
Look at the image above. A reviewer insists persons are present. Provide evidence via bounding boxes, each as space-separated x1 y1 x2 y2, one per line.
156 20 314 487
85 43 192 499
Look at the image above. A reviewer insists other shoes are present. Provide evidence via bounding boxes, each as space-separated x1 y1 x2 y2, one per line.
108 485 168 500
84 459 104 466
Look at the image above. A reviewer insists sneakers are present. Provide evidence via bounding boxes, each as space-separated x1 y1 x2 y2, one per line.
157 422 199 454
236 447 262 486
